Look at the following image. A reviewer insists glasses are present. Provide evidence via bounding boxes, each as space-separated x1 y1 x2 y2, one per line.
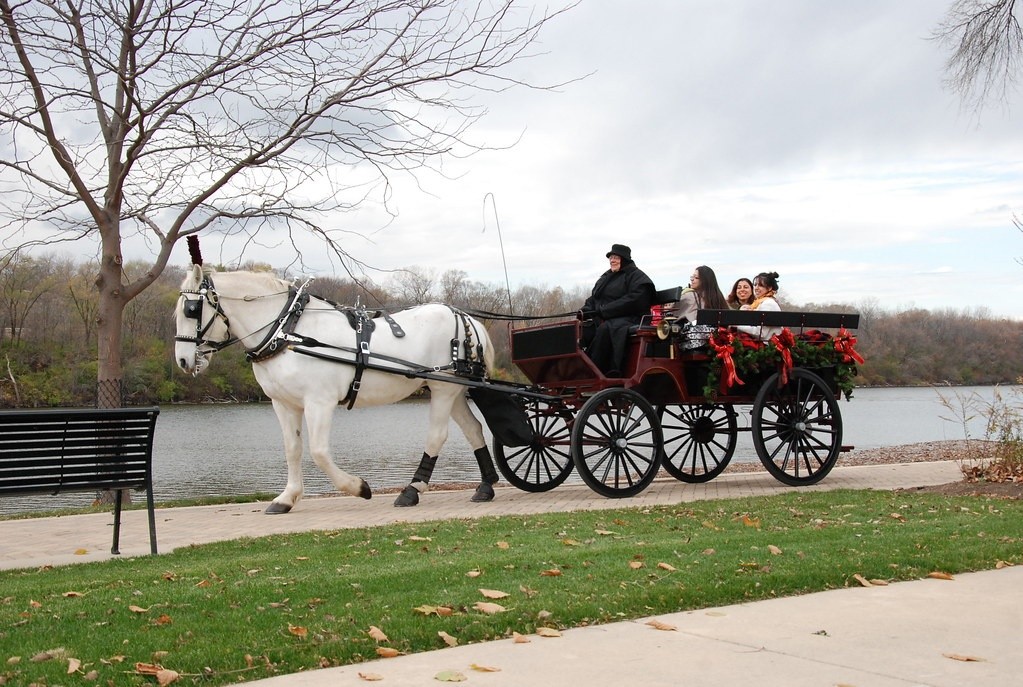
689 275 700 281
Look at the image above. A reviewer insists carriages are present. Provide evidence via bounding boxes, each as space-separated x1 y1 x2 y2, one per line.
171 262 861 516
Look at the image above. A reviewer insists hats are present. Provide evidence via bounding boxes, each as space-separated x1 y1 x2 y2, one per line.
606 244 631 261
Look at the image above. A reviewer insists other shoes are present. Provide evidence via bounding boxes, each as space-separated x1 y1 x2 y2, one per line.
576 369 596 379
606 369 622 378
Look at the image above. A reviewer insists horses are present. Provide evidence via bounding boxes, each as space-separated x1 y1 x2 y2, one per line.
174 264 498 516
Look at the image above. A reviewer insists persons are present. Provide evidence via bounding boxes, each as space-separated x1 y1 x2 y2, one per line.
660 302 673 315
725 278 756 328
577 243 655 378
667 265 728 351
731 272 783 346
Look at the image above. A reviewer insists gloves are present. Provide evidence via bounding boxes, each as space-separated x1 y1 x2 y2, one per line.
577 307 590 322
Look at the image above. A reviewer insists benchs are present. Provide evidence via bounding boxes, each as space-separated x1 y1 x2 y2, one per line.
627 286 687 336
0 406 160 556
